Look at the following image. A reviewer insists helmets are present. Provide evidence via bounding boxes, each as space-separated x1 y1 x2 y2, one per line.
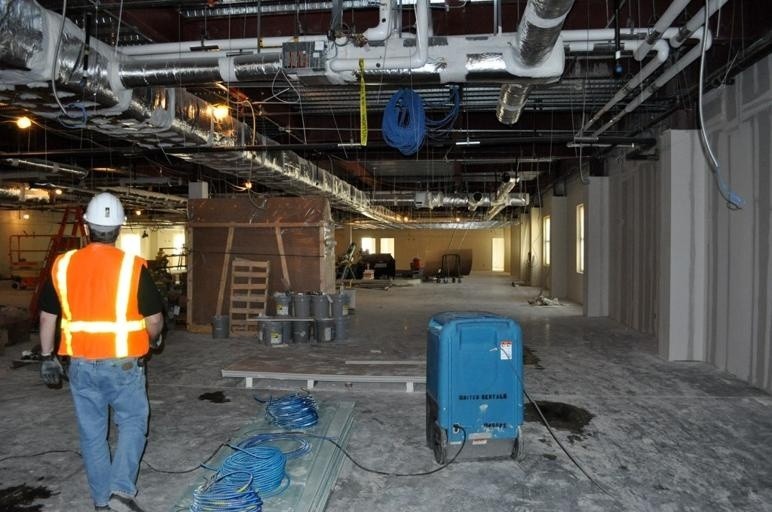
82 192 126 226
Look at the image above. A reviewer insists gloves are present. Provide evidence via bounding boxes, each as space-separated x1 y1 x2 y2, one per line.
150 333 163 350
39 350 65 386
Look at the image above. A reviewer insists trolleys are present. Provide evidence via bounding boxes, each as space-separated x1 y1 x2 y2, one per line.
435 254 464 283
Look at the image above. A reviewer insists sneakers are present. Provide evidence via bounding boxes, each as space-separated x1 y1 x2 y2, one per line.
94 493 145 511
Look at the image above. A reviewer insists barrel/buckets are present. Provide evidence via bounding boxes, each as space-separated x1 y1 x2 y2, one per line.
256 291 350 346
210 315 229 338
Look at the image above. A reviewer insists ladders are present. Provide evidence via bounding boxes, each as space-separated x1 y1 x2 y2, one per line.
29 207 87 328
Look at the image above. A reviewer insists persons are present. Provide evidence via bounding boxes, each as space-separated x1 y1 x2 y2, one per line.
36 189 168 511
